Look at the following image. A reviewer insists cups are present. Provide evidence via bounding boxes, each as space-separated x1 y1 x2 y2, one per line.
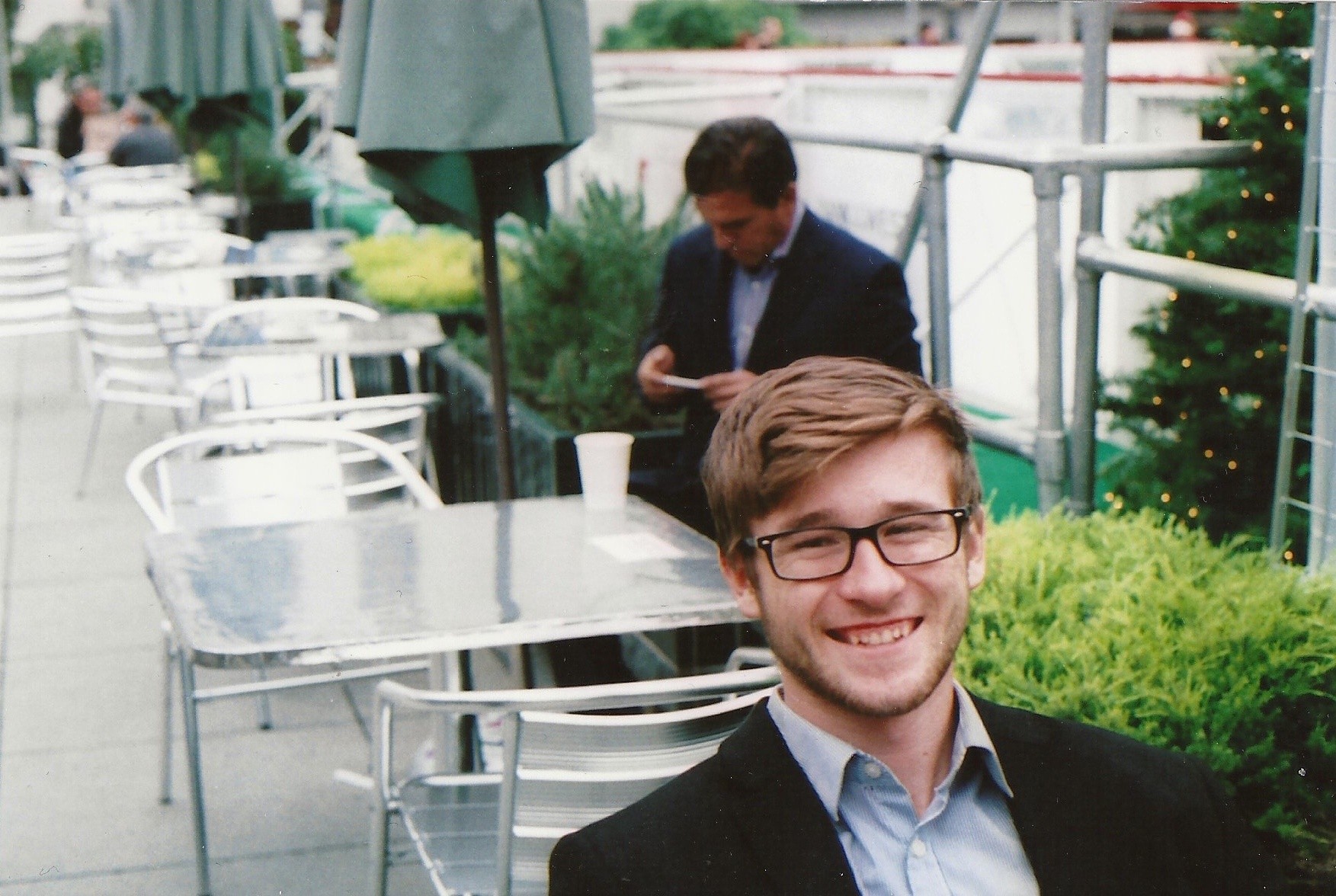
573 432 635 513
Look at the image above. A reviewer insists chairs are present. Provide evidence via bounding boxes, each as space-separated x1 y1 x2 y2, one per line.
0 147 783 896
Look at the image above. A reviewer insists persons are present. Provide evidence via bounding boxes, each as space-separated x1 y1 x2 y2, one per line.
547 357 1282 896
541 115 924 715
110 99 179 168
57 74 102 159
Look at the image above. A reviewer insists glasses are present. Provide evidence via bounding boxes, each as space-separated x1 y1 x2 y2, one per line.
746 504 972 581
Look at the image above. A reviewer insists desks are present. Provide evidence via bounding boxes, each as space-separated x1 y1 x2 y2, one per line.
143 493 761 896
199 312 445 400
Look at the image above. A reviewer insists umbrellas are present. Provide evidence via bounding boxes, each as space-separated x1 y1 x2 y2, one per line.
328 0 595 690
96 0 286 300
